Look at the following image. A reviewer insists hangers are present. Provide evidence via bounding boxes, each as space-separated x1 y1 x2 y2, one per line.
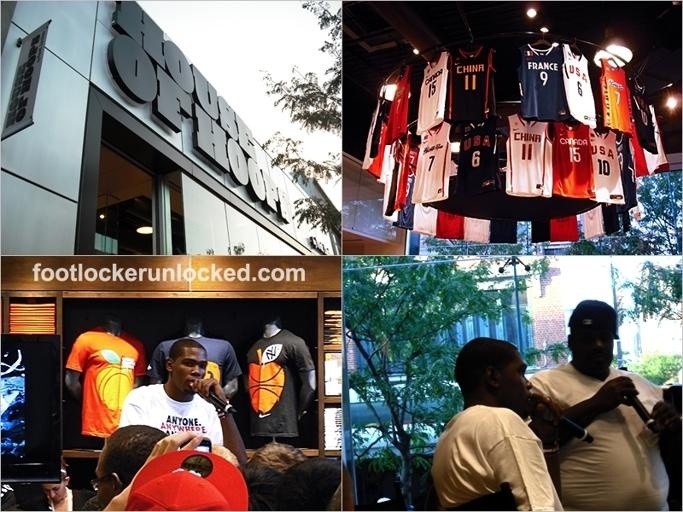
423 31 620 71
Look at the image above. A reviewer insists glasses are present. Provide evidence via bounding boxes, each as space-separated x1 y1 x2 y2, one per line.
91 475 111 491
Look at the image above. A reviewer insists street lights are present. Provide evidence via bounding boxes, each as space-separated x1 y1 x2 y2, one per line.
499 255 531 364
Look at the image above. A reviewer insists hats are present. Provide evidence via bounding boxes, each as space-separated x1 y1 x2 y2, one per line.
125 450 249 511
569 300 620 339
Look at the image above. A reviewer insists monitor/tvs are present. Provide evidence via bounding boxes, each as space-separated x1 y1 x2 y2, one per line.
1 333 62 483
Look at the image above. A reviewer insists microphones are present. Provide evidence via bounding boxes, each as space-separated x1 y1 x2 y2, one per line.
626 394 659 433
190 382 237 414
527 392 593 444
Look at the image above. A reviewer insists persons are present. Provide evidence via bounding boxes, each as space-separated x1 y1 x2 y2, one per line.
0 426 355 510
428 336 564 512
117 338 249 467
528 299 681 511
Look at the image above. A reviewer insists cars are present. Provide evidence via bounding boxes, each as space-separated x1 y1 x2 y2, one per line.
342 372 467 477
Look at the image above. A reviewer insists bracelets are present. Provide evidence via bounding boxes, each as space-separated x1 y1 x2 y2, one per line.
541 441 562 458
216 407 226 419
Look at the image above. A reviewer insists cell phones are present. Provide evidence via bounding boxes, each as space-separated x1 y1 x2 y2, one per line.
180 438 212 453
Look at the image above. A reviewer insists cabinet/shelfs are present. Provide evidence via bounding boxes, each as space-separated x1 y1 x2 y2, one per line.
3 254 344 483
